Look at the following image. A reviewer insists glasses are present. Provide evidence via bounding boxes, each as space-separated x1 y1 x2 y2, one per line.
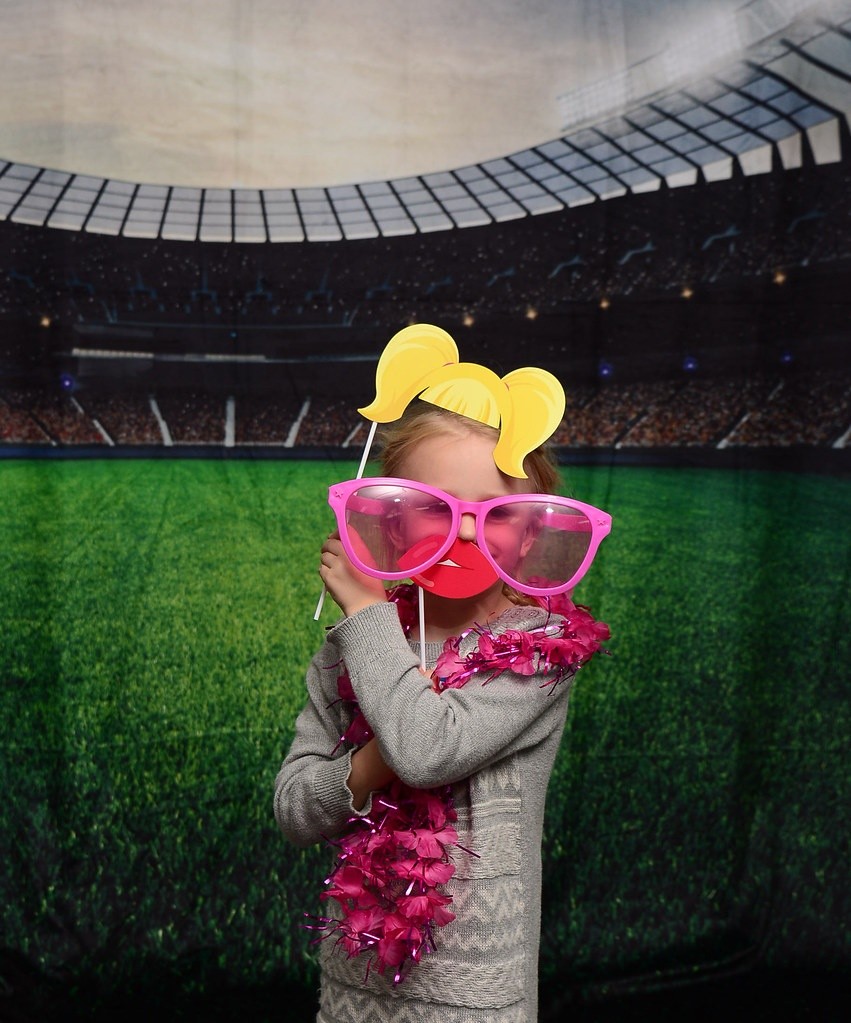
328 477 612 596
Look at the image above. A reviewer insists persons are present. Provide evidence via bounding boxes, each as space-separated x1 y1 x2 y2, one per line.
0 118 850 446
273 410 611 1022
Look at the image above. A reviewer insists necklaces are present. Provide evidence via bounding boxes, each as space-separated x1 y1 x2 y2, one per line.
302 577 609 987
